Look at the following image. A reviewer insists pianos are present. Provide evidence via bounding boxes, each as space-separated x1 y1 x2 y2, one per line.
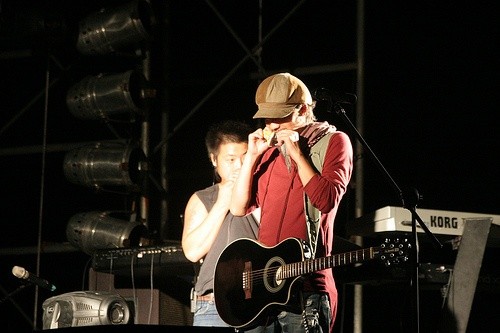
92 245 206 271
352 205 500 235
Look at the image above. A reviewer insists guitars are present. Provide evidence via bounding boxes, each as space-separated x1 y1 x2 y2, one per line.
213 235 415 330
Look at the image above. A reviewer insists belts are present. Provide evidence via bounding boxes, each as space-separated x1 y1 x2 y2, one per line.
197 290 215 301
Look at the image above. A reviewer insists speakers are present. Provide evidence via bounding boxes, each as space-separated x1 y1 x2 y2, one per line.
90 268 194 325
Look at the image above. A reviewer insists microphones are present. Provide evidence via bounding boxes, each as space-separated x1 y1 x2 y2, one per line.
315 88 357 104
12 266 56 291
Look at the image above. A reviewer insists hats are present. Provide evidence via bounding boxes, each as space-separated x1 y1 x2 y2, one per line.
253 71 313 119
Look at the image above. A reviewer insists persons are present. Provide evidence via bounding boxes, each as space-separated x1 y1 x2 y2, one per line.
231 74 353 333
181 128 275 329
